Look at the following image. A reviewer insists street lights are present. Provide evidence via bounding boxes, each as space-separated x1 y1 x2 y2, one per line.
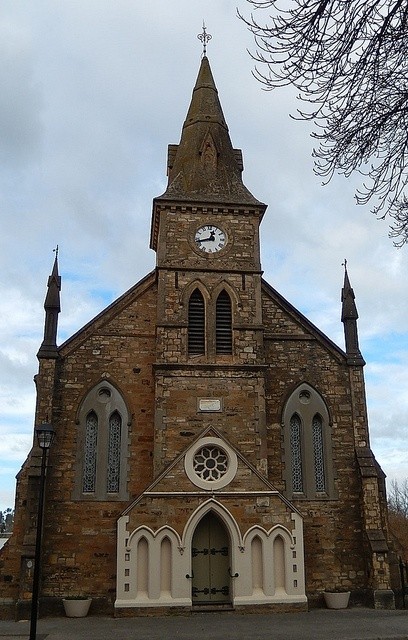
30 414 56 639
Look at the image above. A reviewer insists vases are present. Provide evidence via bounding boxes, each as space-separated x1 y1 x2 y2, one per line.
324 591 350 610
63 597 93 617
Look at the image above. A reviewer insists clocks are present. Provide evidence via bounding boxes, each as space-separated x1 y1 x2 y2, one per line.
187 215 234 259
